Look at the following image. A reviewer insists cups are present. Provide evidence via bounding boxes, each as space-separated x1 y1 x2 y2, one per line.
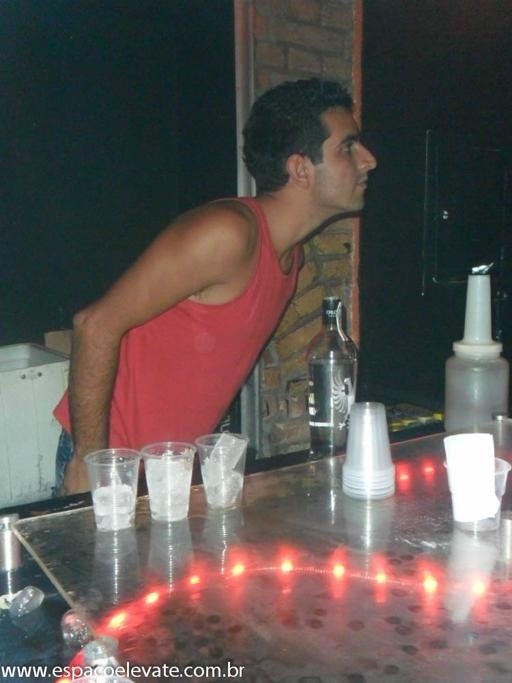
82 448 142 532
443 456 512 532
195 432 249 512
139 441 198 523
341 401 396 501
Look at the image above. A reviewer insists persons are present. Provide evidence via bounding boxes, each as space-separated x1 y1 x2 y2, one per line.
53 75 379 498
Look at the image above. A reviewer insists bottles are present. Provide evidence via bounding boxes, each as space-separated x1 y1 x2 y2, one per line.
444 274 509 434
304 295 359 461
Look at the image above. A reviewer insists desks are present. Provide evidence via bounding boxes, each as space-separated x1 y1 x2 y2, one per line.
11 430 510 681
